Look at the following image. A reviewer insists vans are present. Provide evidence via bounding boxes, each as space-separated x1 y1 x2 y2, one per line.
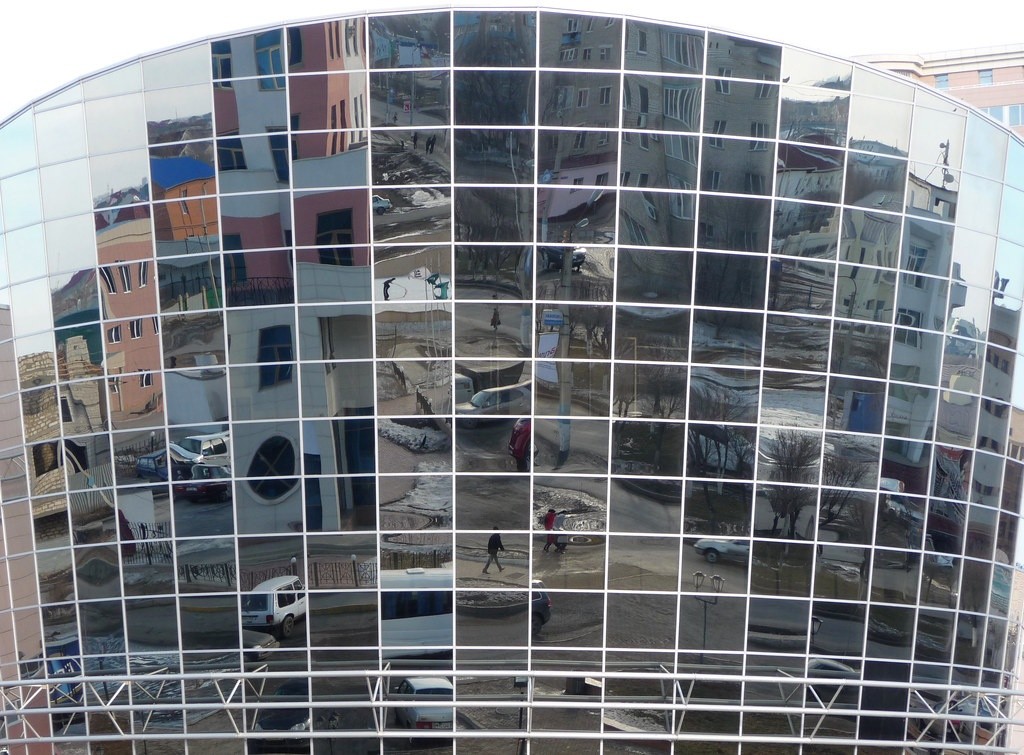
454 372 475 404
395 673 454 741
240 575 306 636
381 566 452 663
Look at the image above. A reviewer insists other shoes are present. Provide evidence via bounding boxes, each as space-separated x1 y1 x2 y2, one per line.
482 568 490 574
499 567 506 572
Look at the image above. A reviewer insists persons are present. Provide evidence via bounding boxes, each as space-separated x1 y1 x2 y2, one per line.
543 508 556 552
482 525 505 574
426 137 431 154
554 510 569 554
429 134 436 154
413 131 418 149
507 418 531 473
491 307 501 330
392 111 398 123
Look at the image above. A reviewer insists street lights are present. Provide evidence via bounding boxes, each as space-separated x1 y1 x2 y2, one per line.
149 431 156 452
408 42 422 128
621 337 639 418
692 570 726 664
351 554 359 589
291 558 298 575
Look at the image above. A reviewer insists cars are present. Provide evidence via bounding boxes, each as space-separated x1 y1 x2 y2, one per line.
817 558 864 591
133 432 232 503
372 195 392 215
455 386 531 429
241 627 282 672
880 459 907 492
917 694 1004 740
693 538 752 563
808 659 858 705
532 578 552 635
540 246 587 273
245 677 337 755
